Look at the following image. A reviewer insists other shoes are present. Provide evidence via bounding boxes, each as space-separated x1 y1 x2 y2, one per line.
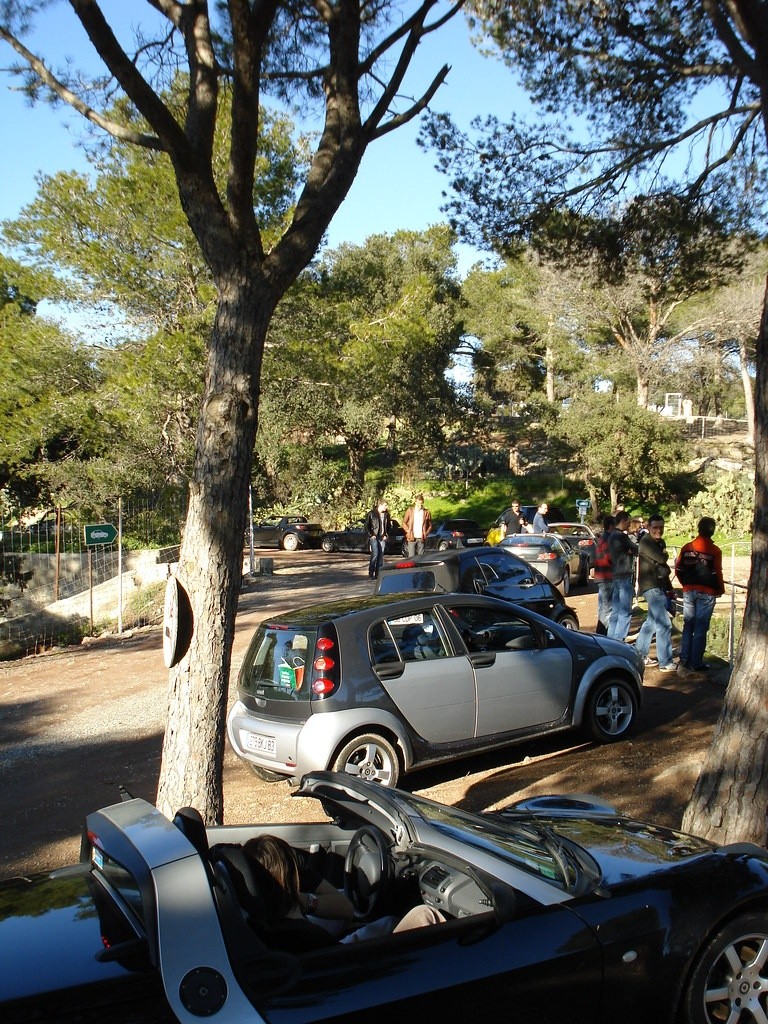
369 574 374 580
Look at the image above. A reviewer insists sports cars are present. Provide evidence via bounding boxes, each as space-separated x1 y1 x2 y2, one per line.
496 532 591 597
0 770 768 1024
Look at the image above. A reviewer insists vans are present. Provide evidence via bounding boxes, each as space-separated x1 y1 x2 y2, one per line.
374 546 580 631
483 504 567 548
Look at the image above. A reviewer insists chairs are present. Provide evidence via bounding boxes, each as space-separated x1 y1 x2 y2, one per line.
176 801 209 849
371 624 395 664
209 845 331 959
402 622 438 660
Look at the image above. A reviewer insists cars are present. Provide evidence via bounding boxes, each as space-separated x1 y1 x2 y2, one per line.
401 518 491 557
224 591 646 790
321 517 409 552
26 508 74 535
546 522 602 570
244 514 325 551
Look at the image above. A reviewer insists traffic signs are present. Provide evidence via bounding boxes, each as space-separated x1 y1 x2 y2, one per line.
84 523 119 547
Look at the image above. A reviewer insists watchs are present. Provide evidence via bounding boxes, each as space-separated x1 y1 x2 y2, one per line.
307 893 318 915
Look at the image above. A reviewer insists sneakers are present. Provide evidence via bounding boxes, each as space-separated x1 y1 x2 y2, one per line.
660 662 680 672
644 657 658 666
678 664 714 674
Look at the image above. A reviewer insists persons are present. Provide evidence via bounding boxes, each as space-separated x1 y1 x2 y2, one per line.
403 495 433 558
595 504 650 646
365 498 391 582
675 517 725 671
242 835 448 956
635 516 680 673
500 499 551 541
